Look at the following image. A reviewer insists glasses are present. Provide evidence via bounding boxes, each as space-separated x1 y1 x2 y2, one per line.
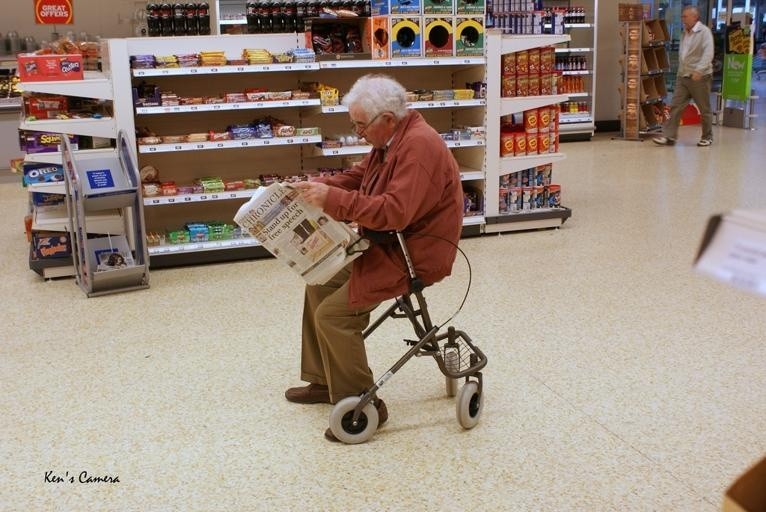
348 115 379 139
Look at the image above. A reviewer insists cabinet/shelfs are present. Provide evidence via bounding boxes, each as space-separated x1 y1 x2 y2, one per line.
13 1 671 298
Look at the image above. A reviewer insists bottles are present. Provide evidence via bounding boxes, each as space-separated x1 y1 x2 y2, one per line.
543 6 586 24
561 100 587 112
146 0 211 37
246 0 371 33
556 56 588 70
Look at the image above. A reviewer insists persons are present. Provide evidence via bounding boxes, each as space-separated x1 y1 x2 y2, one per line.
651 4 715 146
285 71 466 442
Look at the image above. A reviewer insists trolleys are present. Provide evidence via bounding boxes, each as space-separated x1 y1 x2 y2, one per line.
329 224 488 445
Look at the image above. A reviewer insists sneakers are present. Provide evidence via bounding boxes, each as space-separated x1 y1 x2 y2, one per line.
324 399 389 442
652 136 675 145
697 138 713 146
285 383 336 407
